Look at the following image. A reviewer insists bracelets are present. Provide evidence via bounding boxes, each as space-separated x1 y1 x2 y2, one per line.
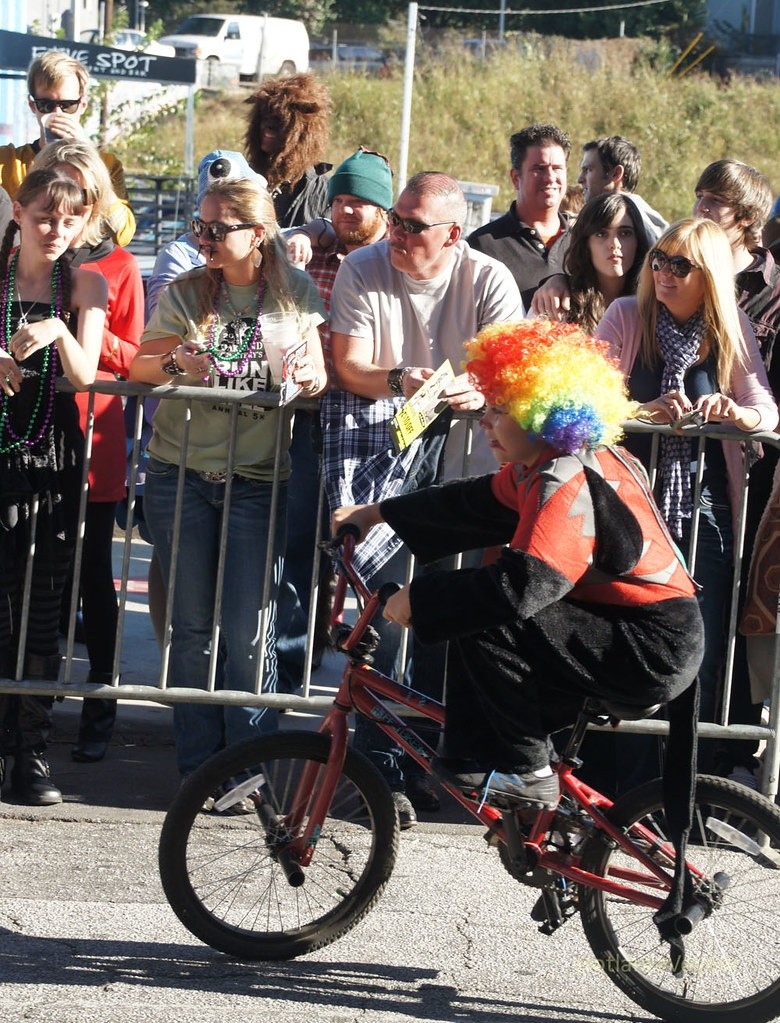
162 344 188 378
293 228 312 238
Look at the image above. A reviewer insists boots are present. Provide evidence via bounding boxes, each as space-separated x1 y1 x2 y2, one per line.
71 670 121 761
10 649 63 806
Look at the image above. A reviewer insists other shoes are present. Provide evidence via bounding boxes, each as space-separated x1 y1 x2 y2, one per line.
203 793 219 815
227 787 264 815
59 611 89 644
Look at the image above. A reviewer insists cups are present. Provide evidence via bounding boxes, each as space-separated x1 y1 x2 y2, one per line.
257 313 299 386
40 111 64 144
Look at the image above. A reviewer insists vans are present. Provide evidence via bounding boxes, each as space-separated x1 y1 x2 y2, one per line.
307 45 388 76
158 13 310 80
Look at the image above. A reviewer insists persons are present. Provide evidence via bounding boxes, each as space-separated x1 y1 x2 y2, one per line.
0 185 20 252
390 368 404 398
276 145 393 694
241 75 331 713
465 126 780 846
116 149 337 706
0 51 136 248
130 181 329 815
331 314 705 922
328 173 526 828
25 138 144 762
0 169 108 808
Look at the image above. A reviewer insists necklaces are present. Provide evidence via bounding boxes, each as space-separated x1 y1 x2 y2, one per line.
0 248 61 452
194 269 266 384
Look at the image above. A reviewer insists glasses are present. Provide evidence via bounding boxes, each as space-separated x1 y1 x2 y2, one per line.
30 94 82 114
648 250 703 278
386 207 456 233
191 217 252 242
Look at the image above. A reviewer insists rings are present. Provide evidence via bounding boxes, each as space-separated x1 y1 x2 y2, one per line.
5 370 14 381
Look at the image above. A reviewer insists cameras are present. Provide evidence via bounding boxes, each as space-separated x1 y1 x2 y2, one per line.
670 405 707 429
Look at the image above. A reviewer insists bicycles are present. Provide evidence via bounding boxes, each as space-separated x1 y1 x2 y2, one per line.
155 527 780 1023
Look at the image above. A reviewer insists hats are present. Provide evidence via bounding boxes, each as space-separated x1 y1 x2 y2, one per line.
194 149 269 208
326 147 393 212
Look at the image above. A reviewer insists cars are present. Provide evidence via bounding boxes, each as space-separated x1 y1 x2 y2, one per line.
79 27 174 58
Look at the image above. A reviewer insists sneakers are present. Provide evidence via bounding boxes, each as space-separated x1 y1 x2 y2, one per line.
432 755 560 812
401 773 439 811
359 791 417 828
